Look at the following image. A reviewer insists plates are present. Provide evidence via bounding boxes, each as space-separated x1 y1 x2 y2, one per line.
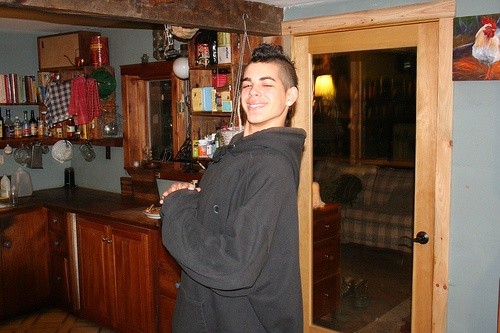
143 207 161 219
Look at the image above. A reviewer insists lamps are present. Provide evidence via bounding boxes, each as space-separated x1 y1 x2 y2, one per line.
315 75 336 97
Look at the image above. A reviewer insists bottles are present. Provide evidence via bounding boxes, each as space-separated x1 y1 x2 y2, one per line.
142 52 148 62
193 132 220 158
0 173 11 199
0 107 4 139
216 94 222 112
4 108 14 139
165 44 179 61
29 110 38 138
210 40 218 64
38 112 98 140
11 166 33 197
31 138 38 146
98 104 123 141
13 115 22 139
22 111 31 137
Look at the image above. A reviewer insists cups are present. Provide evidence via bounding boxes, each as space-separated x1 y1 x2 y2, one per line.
38 142 49 154
8 184 19 208
79 142 96 162
3 144 13 158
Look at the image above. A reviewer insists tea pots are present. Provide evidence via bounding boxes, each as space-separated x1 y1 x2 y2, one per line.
64 48 91 68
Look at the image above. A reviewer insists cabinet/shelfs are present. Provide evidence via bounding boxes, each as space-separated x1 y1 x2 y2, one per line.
0 0 283 333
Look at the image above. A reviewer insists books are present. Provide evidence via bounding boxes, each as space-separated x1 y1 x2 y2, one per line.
0 73 36 104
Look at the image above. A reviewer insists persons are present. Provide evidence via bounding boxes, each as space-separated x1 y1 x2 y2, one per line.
159 43 304 333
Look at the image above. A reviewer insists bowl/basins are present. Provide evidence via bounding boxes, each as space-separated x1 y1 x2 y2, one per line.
142 161 162 169
